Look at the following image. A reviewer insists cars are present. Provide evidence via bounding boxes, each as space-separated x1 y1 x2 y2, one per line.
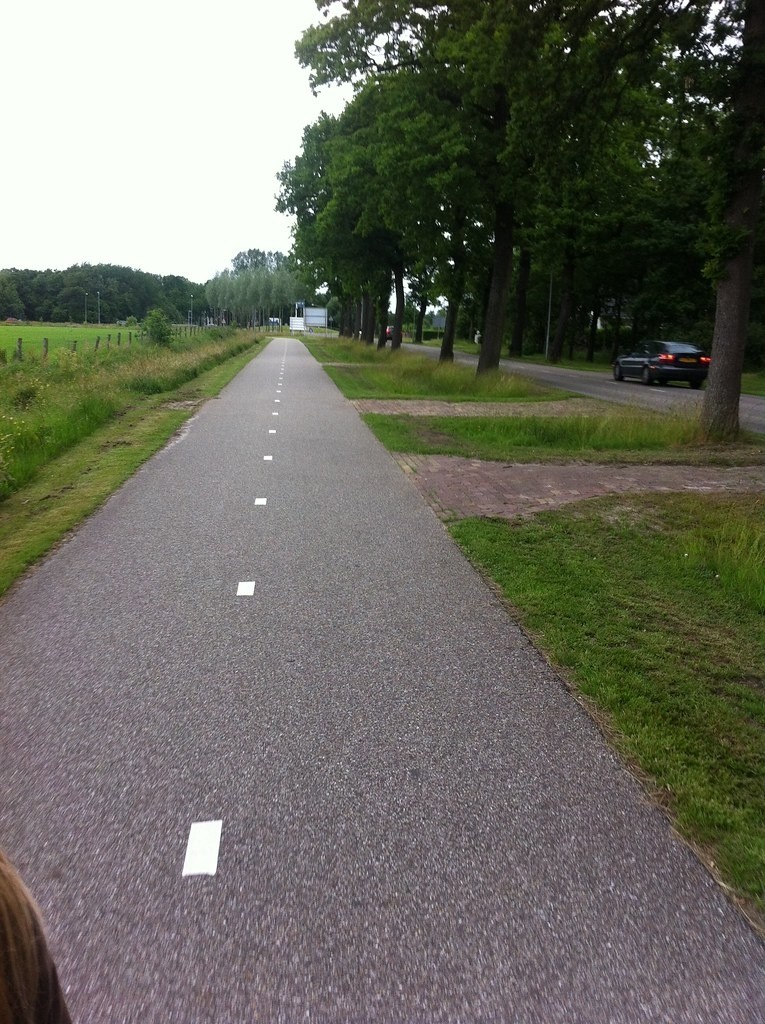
609 340 710 388
384 325 401 341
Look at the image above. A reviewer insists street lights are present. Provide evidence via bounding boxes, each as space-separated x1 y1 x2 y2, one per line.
83 292 90 324
190 294 193 324
96 292 102 324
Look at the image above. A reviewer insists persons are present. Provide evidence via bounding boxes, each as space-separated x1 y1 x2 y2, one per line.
0 850 74 1024
